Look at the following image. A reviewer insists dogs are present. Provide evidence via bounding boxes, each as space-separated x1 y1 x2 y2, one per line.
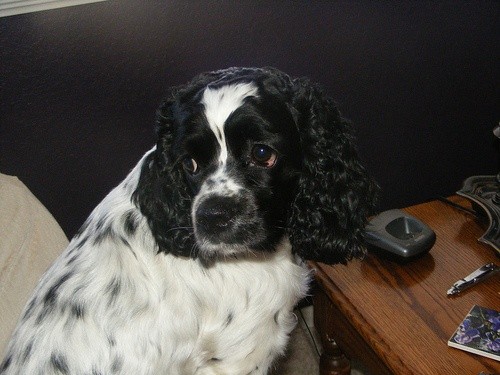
0 64 372 375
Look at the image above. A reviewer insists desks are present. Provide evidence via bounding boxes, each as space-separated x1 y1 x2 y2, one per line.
305 195 500 375
